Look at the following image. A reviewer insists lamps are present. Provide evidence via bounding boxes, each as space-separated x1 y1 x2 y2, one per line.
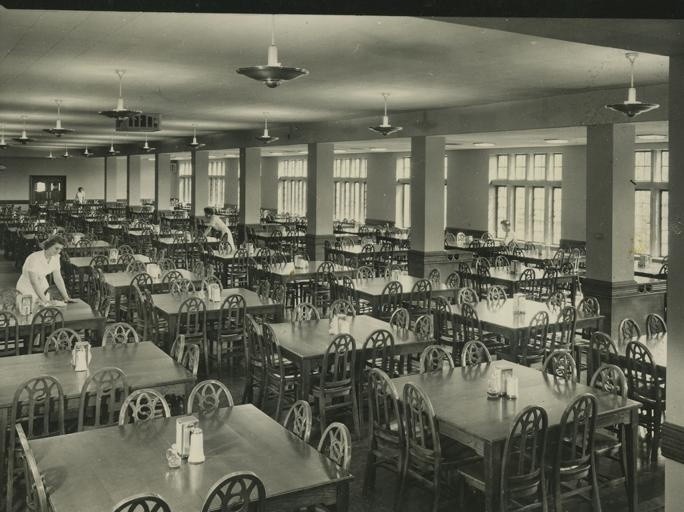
107 132 120 156
235 15 309 88
98 69 143 122
139 135 156 152
0 99 93 171
368 93 403 137
604 52 660 119
255 112 279 144
185 128 206 150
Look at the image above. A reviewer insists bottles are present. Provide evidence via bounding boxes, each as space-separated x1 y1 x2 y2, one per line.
296 256 304 268
74 346 88 373
339 316 349 334
211 286 220 302
392 269 399 281
187 428 206 466
520 297 526 315
19 296 32 317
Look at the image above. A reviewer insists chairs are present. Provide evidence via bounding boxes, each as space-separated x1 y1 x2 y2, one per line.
0 197 669 511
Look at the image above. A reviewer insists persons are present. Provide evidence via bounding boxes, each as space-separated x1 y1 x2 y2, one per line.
15 236 77 313
71 187 85 211
498 218 517 247
201 207 235 250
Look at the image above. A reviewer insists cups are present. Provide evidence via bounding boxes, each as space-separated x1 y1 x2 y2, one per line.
504 376 519 400
